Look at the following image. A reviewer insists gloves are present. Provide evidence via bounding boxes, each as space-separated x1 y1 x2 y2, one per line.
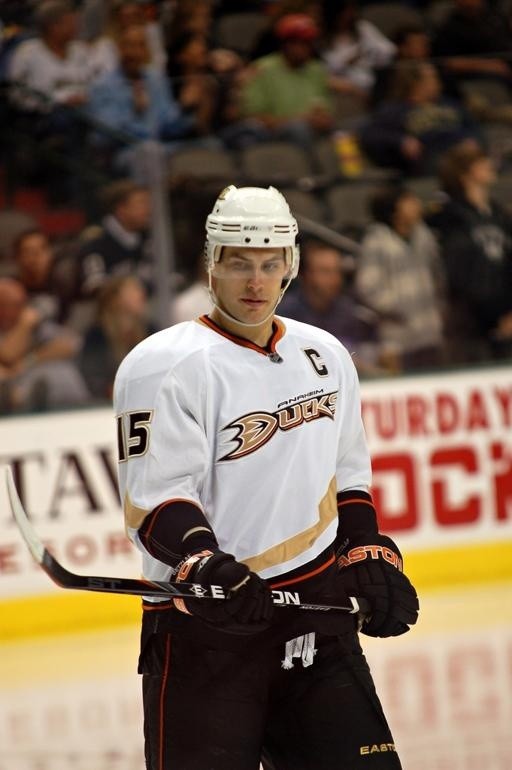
170 545 279 636
332 524 419 639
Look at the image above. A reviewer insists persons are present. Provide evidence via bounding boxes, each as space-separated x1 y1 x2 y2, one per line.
111 185 420 769
0 2 511 418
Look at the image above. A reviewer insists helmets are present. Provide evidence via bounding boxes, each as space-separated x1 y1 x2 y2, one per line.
203 184 301 281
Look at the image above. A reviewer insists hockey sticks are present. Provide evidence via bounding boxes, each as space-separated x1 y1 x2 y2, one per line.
6 465 371 613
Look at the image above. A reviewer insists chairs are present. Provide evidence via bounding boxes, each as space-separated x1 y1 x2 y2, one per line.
160 128 392 237
